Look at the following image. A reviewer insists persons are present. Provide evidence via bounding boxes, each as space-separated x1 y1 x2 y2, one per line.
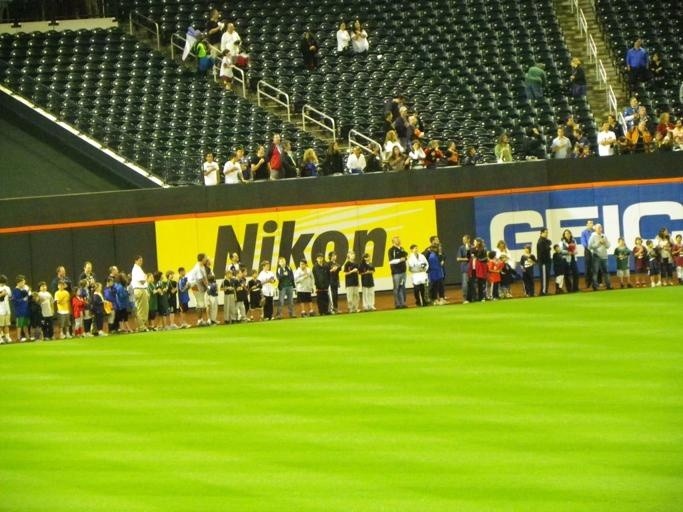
187 253 376 327
388 236 450 309
0 253 192 345
456 220 682 304
197 9 682 187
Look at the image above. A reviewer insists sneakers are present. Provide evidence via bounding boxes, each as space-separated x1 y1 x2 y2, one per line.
462 293 512 304
620 280 672 289
0 323 190 344
195 306 376 327
396 298 449 310
